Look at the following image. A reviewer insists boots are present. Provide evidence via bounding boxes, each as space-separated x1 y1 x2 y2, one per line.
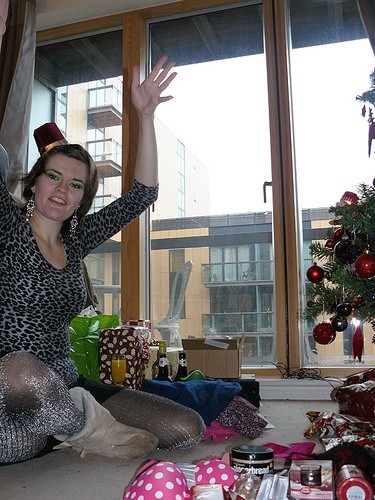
52 387 159 460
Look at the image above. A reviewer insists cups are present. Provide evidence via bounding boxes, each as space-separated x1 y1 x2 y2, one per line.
231 445 274 479
112 354 126 381
300 464 322 486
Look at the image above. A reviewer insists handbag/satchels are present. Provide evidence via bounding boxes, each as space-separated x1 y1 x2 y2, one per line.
68 305 119 382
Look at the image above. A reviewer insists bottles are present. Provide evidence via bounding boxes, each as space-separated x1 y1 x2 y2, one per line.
174 350 188 381
335 464 373 500
152 340 173 380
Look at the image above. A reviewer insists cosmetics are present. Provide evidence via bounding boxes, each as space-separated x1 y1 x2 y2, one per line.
230 445 274 476
335 467 374 500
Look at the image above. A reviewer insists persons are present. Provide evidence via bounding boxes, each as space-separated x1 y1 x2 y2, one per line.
0 55 206 463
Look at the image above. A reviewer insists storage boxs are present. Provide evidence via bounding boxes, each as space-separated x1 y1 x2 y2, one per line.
288 459 334 500
99 325 150 390
181 334 246 379
